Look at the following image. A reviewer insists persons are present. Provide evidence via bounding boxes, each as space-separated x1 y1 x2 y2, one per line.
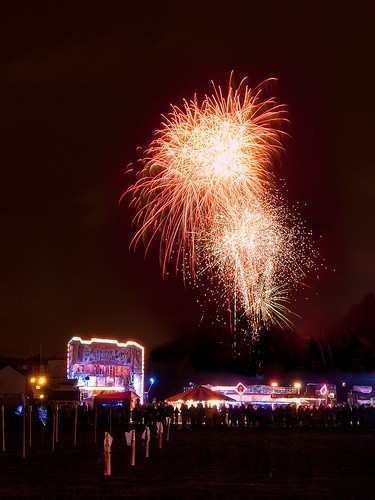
27 401 375 431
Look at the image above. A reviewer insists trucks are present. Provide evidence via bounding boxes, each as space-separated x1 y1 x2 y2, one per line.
28 379 84 420
346 385 373 408
